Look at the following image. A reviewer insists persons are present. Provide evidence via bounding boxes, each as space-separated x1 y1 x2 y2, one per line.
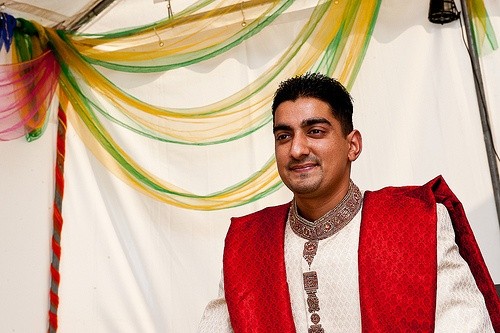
193 73 500 333
